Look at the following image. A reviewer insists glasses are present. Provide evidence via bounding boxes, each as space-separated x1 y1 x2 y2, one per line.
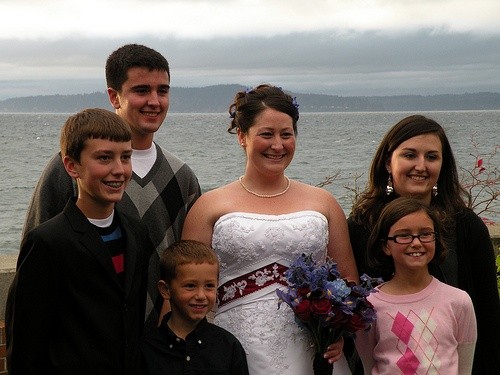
386 232 439 244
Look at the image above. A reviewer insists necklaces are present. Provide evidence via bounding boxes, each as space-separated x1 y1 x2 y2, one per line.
238 175 292 198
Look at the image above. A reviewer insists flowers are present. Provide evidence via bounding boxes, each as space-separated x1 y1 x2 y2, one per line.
275 252 383 375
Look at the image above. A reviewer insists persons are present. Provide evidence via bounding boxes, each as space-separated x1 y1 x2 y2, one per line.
4 108 148 375
348 115 500 375
19 42 202 337
353 196 477 375
133 239 250 375
160 81 359 375
